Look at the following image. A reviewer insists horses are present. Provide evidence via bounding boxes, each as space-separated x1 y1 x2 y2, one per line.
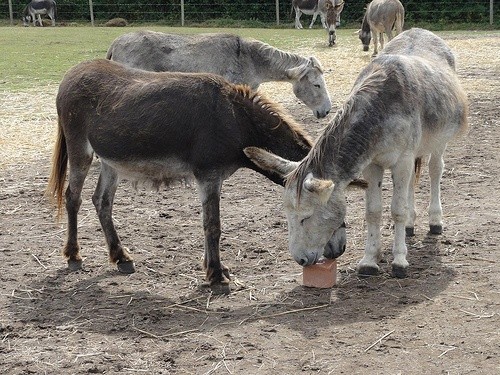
50 0 468 295
21 0 57 27
